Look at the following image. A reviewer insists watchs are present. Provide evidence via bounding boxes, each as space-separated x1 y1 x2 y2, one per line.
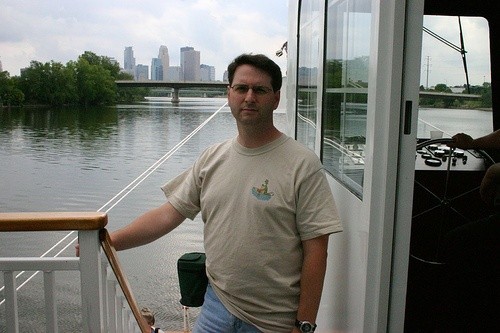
295 320 317 333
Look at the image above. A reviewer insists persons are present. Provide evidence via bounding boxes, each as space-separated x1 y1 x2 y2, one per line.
140 307 164 333
77 55 342 333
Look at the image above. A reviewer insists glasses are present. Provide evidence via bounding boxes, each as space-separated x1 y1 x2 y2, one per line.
230 83 275 97
